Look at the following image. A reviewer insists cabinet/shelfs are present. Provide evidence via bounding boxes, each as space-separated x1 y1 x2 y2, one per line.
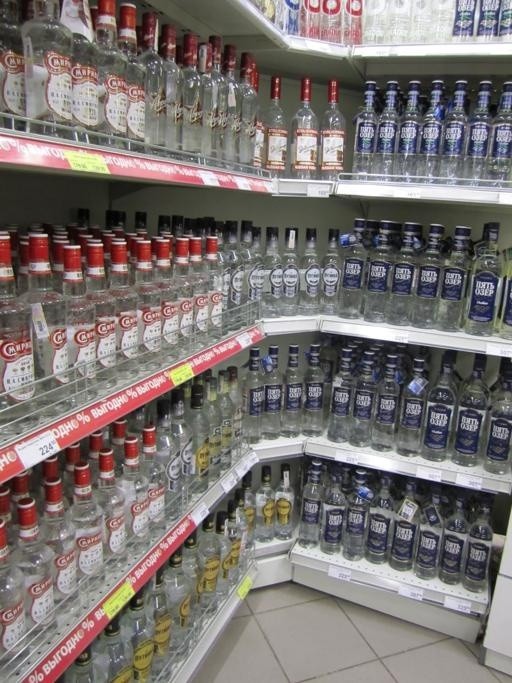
0 0 512 678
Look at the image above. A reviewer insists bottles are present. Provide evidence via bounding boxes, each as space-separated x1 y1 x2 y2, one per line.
62 441 81 506
0 234 40 432
497 0 512 42
263 345 283 441
328 357 353 443
386 0 411 45
255 465 275 543
318 228 343 315
224 221 245 331
413 491 444 581
179 33 202 162
342 0 362 47
321 332 337 416
483 379 512 474
282 344 302 438
172 238 192 355
464 79 495 186
223 44 243 171
0 208 215 278
94 1 129 151
352 80 378 180
394 81 421 182
411 1 431 44
301 344 326 437
156 399 182 520
234 488 249 571
85 430 104 485
97 618 134 683
365 476 394 564
20 0 73 142
107 242 141 380
362 0 386 45
186 384 208 493
464 222 501 337
237 52 257 174
453 0 476 43
0 486 18 553
218 370 233 469
0 1 26 133
203 377 221 480
370 363 396 453
438 496 469 585
199 513 221 615
431 0 453 44
0 519 29 662
461 495 493 593
434 226 471 333
119 587 154 683
299 228 320 316
417 80 445 182
136 11 171 157
35 454 69 518
336 218 368 319
92 449 127 557
317 78 348 180
451 368 488 467
411 224 445 330
128 404 152 448
197 42 220 164
499 247 512 340
226 498 240 586
438 79 469 184
263 226 281 319
19 235 71 415
348 360 377 448
299 460 324 549
239 346 263 444
13 469 41 533
483 80 511 186
62 245 98 401
337 338 512 391
69 461 105 591
477 0 500 44
64 645 106 683
109 419 131 476
386 222 419 326
263 75 288 177
212 222 228 336
41 476 77 608
85 243 118 389
320 471 346 555
164 548 190 662
372 79 399 182
60 1 100 145
242 470 254 547
320 0 342 43
190 237 208 348
228 366 243 445
145 568 173 683
182 529 203 636
343 469 370 561
363 220 395 323
288 76 319 181
117 3 151 152
116 437 149 543
239 220 255 327
397 358 429 458
274 463 294 540
281 228 299 316
209 35 229 169
203 236 222 338
216 510 229 599
11 497 58 643
299 0 320 40
157 24 184 158
171 388 192 505
421 363 457 462
254 70 263 174
142 425 167 528
154 239 179 362
389 482 421 571
131 240 161 371
252 228 263 322
